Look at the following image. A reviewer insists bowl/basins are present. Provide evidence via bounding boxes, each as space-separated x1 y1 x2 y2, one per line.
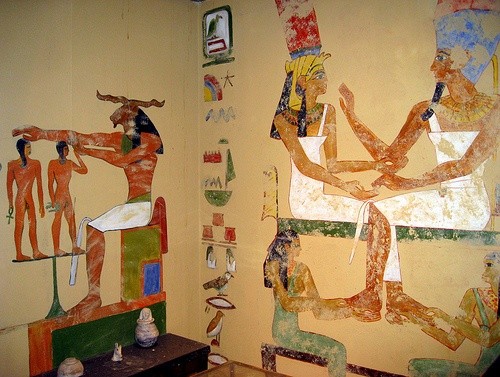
57 357 84 377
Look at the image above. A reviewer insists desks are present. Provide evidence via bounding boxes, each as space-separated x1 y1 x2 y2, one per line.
34 332 212 377
